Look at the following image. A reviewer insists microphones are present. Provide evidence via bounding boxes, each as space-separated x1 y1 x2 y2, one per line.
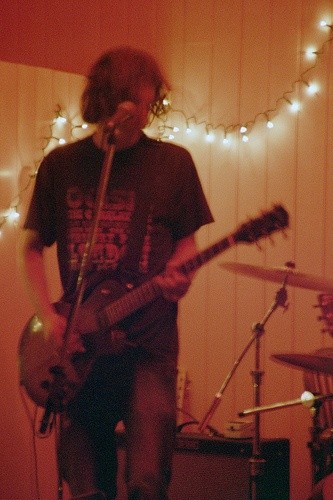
102 101 137 133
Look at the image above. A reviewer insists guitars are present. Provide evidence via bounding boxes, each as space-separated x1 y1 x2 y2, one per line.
19 203 292 408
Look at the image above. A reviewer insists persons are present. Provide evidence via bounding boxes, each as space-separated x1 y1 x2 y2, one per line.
22 46 215 500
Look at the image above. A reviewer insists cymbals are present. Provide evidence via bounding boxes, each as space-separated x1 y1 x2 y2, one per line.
268 353 333 374
218 259 332 290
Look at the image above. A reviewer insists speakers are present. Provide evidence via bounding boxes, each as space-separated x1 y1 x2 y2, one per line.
117 430 291 500
